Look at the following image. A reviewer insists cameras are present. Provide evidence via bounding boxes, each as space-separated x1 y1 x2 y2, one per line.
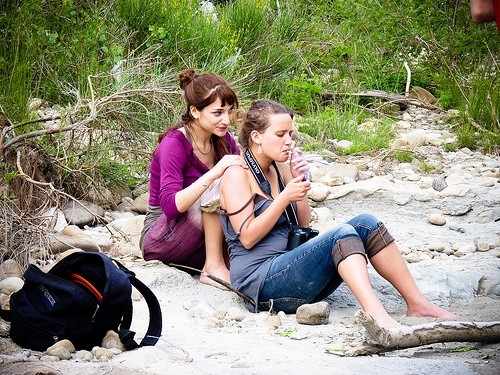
286 227 319 251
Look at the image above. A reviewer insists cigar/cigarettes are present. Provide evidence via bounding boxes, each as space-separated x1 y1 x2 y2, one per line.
287 151 305 157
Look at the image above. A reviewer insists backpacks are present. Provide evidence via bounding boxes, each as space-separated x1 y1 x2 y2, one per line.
10 251 163 352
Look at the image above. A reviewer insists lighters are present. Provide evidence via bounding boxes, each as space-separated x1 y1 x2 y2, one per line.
302 175 306 182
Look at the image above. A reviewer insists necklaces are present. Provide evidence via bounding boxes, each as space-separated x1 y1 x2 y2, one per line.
189 131 212 154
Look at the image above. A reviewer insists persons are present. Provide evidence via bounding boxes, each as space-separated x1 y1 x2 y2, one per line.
138 68 250 291
216 98 461 328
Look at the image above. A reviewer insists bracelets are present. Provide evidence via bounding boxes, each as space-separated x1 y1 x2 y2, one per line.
198 178 209 190
